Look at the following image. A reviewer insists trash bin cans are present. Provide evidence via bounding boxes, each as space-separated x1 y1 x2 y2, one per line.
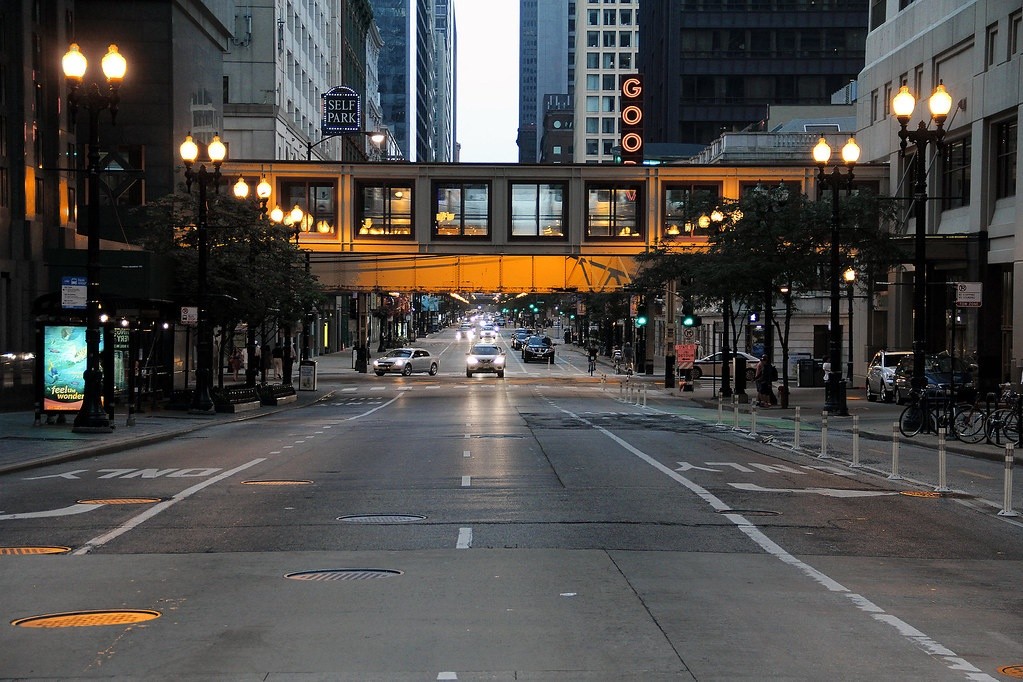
299 360 317 391
814 359 826 387
797 358 814 387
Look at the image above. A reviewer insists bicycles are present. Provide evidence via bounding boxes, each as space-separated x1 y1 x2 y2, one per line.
898 387 1023 449
586 355 599 376
612 359 633 378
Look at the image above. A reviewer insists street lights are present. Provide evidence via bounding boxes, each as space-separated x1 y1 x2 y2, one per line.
844 266 854 387
698 204 734 397
892 78 953 434
271 202 303 383
61 40 127 434
811 133 860 417
510 328 555 364
179 130 226 415
233 172 272 409
303 131 386 357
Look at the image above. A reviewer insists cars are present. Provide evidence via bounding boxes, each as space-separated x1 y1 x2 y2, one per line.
373 347 441 376
865 347 938 404
676 351 762 381
429 314 503 340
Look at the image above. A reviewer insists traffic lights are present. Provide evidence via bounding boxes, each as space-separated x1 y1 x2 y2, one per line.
568 310 576 321
749 312 757 323
501 304 540 315
558 310 565 315
680 315 702 327
637 303 649 327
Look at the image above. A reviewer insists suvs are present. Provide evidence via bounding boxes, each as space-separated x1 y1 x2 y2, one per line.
892 354 979 406
465 343 507 378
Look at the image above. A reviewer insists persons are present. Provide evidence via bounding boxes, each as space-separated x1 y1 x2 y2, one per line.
255 340 296 380
612 343 633 374
213 341 219 375
230 343 248 382
585 344 598 372
695 341 703 360
823 358 831 380
755 354 766 405
